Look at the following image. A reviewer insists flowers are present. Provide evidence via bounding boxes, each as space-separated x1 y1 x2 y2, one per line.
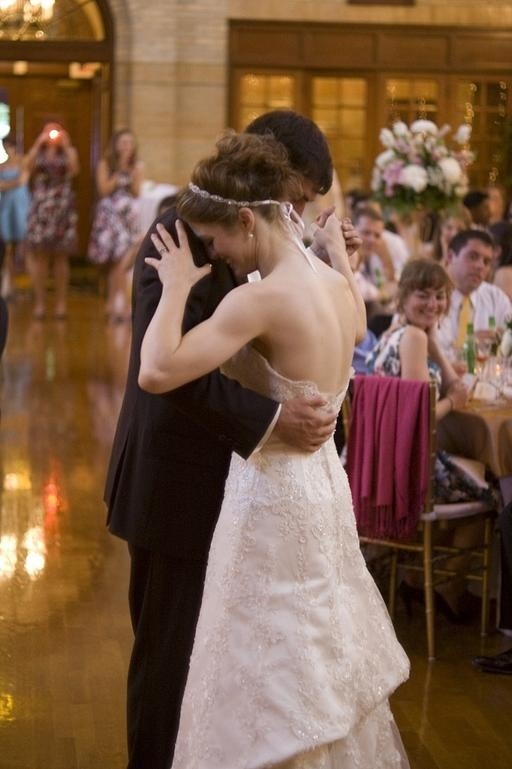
364 115 483 218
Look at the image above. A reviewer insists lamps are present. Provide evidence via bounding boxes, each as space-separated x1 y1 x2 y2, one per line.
0 0 62 45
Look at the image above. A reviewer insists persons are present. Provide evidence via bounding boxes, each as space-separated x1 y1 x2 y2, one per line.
21 123 79 319
473 423 512 674
340 186 512 256
391 230 511 367
341 209 398 339
104 110 363 767
0 134 32 301
137 131 411 769
364 257 500 627
88 128 143 321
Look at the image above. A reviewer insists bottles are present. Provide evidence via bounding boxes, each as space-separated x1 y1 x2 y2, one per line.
486 316 499 358
463 323 476 375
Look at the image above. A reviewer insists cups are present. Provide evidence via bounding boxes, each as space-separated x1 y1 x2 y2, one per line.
473 340 493 382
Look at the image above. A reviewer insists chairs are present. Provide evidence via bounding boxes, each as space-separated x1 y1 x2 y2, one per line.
341 377 499 667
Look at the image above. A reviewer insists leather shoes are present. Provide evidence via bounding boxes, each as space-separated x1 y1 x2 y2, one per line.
473 648 512 673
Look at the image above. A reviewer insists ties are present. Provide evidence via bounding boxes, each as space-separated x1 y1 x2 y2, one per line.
362 256 374 281
454 296 469 347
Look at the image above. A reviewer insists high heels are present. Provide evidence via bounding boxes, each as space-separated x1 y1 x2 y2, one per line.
400 581 424 619
435 590 465 624
